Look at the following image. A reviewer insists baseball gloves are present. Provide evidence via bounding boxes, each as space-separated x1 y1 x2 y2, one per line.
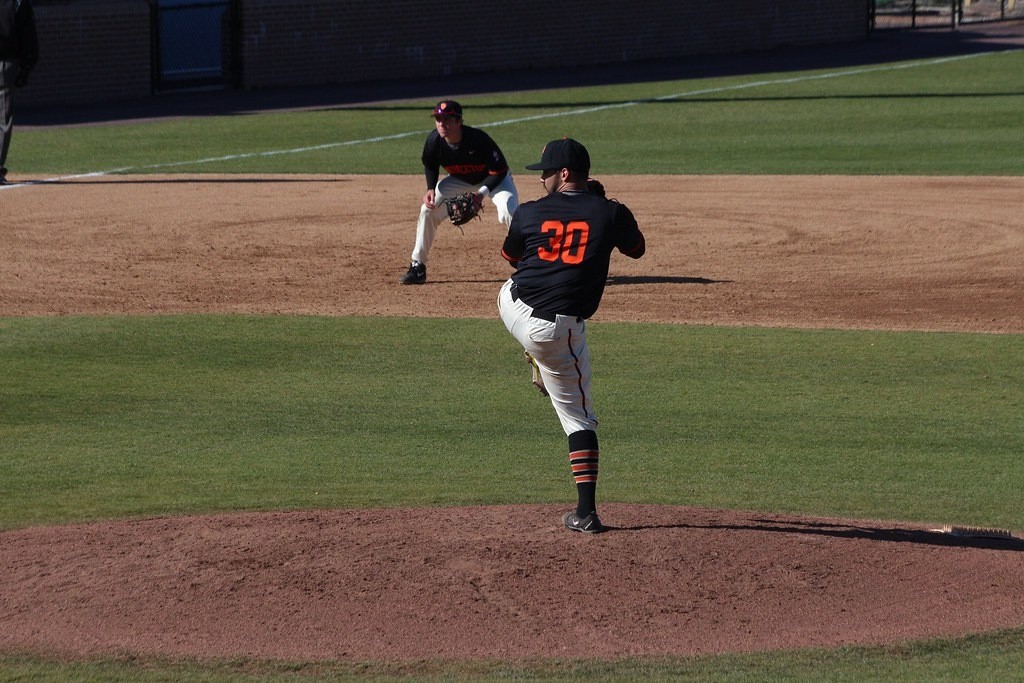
586 178 607 200
445 192 483 226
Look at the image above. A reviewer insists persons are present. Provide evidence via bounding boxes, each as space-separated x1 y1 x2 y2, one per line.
397 99 517 285
0 0 40 185
496 138 646 534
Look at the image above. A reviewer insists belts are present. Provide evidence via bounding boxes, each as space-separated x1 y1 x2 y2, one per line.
511 281 582 324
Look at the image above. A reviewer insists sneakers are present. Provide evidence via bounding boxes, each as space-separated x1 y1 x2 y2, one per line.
523 350 548 396
398 262 426 284
562 510 602 533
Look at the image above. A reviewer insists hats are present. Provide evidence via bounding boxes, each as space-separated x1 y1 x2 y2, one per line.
430 100 463 118
525 136 591 180
